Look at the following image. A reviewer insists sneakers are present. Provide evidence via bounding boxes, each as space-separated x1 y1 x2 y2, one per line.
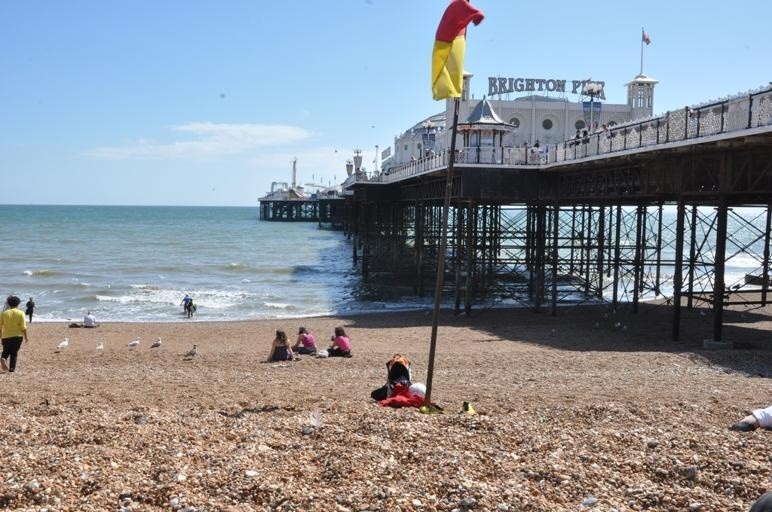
0 358 9 371
419 404 444 414
462 401 475 415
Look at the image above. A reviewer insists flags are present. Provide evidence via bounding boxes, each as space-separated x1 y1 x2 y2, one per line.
431 0 485 101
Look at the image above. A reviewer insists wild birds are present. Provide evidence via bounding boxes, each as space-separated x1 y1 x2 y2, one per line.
593 305 631 333
700 310 708 317
126 336 141 347
149 337 162 349
184 344 197 359
95 341 104 352
56 337 69 352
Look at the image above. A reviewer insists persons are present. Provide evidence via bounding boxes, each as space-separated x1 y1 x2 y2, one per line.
291 325 318 354
187 298 195 318
83 310 95 328
533 140 540 148
575 123 609 145
180 294 191 316
0 296 30 372
728 403 772 512
25 297 38 323
266 328 294 362
326 325 353 357
